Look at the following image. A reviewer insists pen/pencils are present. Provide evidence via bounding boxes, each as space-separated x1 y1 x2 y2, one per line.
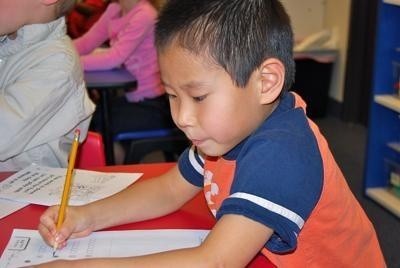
53 128 80 256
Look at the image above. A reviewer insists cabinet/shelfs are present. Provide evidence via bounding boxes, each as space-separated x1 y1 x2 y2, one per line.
362 0 400 220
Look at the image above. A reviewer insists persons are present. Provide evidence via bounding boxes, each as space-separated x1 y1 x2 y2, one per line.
18 0 392 267
71 0 176 167
1 1 99 174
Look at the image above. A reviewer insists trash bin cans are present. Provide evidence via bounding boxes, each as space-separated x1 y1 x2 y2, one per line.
292 39 337 120
